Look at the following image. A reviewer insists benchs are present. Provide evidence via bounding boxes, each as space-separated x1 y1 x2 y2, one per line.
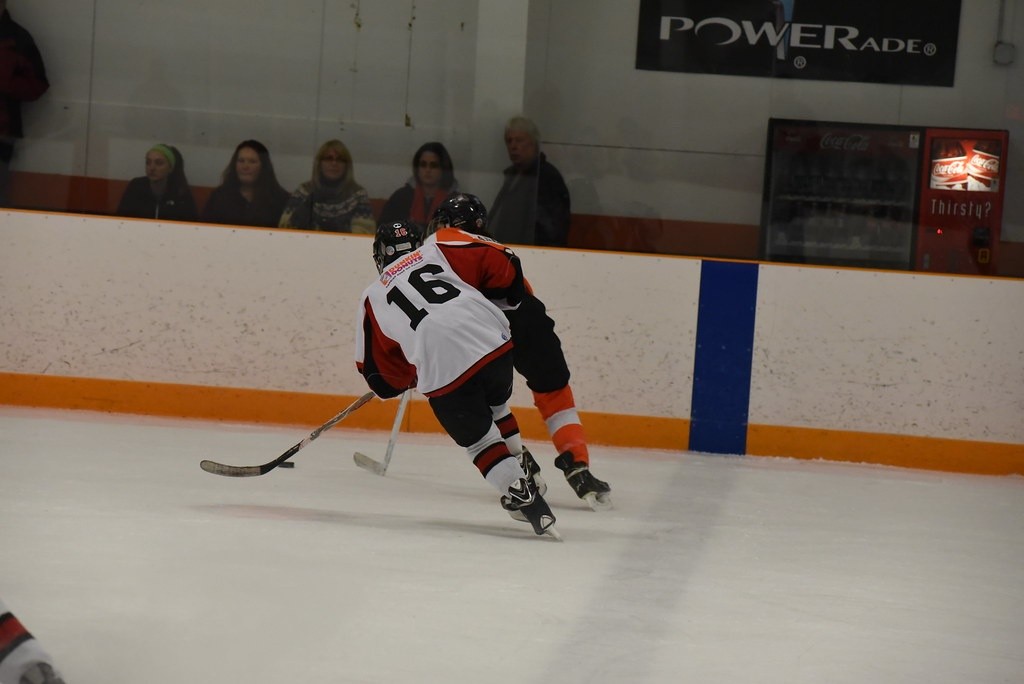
0 168 761 265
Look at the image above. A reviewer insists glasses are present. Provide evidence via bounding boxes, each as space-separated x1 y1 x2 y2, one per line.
419 160 439 169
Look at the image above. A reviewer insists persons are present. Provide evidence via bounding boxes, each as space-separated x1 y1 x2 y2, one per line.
196 140 292 228
425 192 613 511
0 601 70 684
485 117 571 246
374 136 459 236
0 0 49 210
117 146 197 223
278 138 373 236
357 218 563 542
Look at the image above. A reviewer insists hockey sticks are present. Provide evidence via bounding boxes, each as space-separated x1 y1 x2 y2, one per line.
199 389 410 478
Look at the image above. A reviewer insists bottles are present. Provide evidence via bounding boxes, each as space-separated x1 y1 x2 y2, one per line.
930 135 968 193
967 137 1003 192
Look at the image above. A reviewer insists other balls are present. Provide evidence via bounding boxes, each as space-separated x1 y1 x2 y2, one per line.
278 461 295 468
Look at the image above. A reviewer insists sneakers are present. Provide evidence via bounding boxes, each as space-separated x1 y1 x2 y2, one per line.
520 444 547 495
508 478 563 542
499 454 538 522
555 451 614 512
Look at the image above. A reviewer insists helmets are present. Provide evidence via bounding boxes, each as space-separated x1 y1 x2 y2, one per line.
426 192 487 237
373 218 424 275
